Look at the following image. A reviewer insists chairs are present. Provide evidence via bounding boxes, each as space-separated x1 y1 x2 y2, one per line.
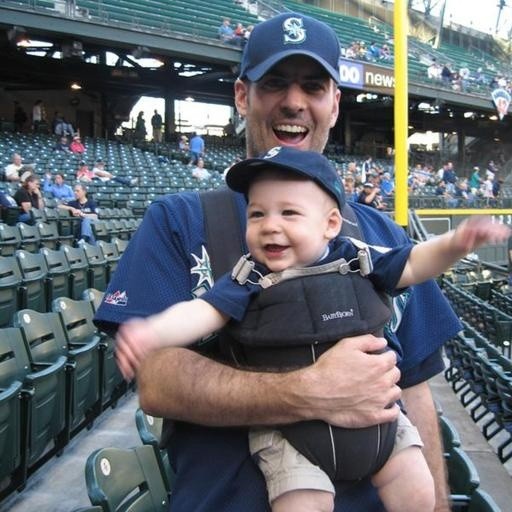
0 121 512 512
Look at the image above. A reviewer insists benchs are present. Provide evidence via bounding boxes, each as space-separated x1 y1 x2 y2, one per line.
0 0 512 98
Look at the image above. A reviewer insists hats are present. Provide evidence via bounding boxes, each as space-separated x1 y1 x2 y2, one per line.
237 12 341 90
226 147 345 214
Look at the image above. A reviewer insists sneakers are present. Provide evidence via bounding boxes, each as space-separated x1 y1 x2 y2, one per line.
77 239 85 247
129 177 138 186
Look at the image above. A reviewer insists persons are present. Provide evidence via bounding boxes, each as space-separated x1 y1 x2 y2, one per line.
217 15 511 101
505 235 512 289
329 140 512 214
91 10 465 510
1 153 75 225
76 160 102 182
56 183 102 249
92 159 139 188
151 109 162 144
178 134 189 150
12 100 85 153
135 110 148 141
191 161 210 182
225 117 237 138
187 132 205 168
110 146 512 511
